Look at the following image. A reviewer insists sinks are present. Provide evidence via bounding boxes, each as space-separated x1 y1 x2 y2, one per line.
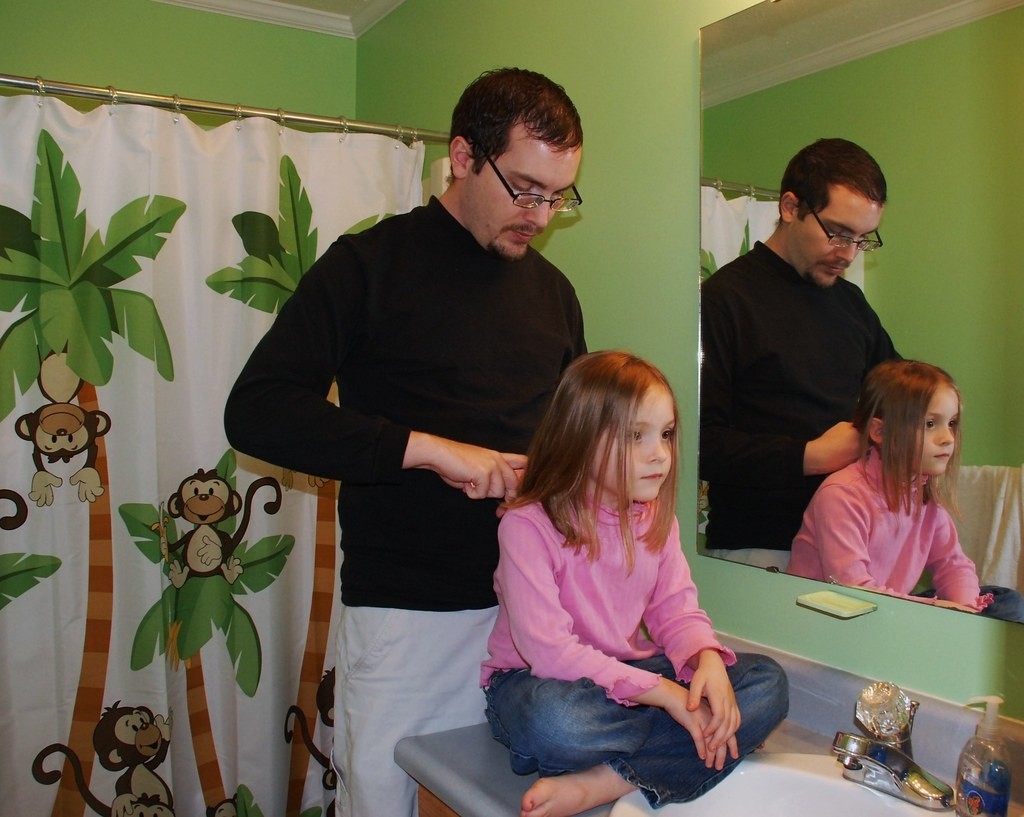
610 754 959 816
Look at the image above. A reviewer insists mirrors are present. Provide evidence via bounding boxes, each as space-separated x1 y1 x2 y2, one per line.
699 0 1024 628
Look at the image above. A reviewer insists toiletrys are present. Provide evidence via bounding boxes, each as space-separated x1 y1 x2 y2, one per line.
956 696 1012 816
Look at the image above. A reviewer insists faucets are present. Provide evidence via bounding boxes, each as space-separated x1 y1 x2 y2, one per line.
832 682 953 810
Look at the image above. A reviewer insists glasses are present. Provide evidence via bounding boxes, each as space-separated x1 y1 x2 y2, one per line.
469 137 581 212
801 196 884 250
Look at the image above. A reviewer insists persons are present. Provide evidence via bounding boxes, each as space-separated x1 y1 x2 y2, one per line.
223 67 591 817
787 361 1024 624
699 136 901 572
479 345 791 816
